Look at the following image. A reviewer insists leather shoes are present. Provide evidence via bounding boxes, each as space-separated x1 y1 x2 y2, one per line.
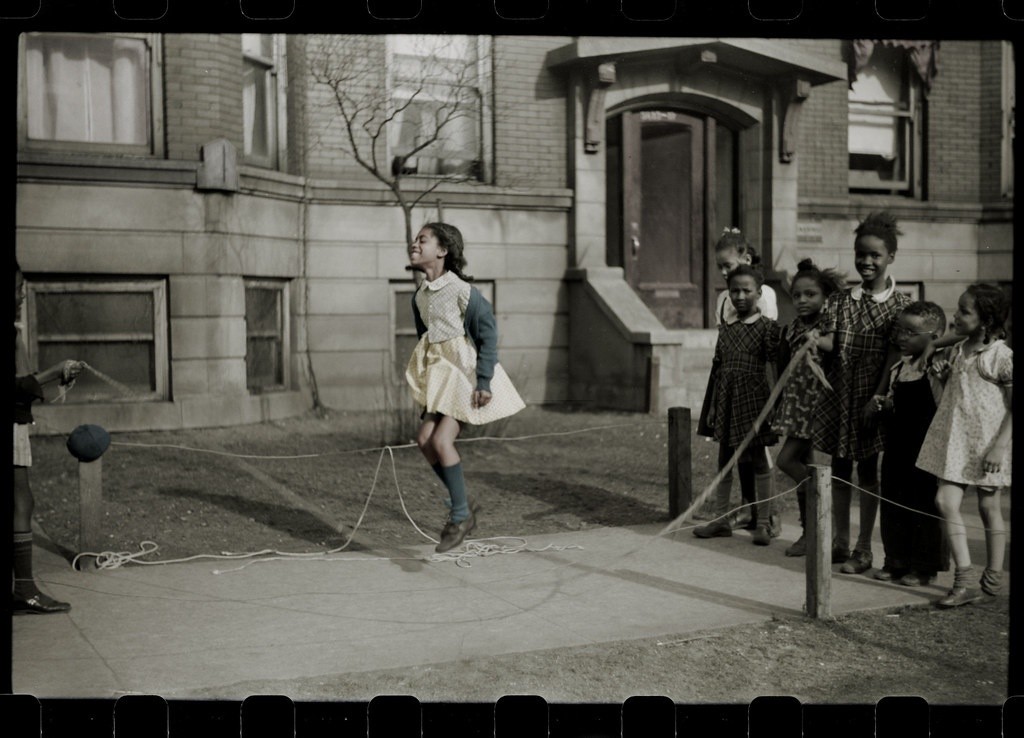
435 512 475 553
441 499 482 538
12 593 72 616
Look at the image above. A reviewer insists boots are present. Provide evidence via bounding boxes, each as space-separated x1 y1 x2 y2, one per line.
969 568 1003 604
939 565 984 606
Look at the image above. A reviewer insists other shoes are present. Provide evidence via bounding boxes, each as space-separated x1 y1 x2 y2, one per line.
769 523 783 537
900 570 920 587
729 509 754 531
840 549 873 573
874 569 891 580
786 536 807 556
693 521 732 537
832 547 850 563
753 528 769 544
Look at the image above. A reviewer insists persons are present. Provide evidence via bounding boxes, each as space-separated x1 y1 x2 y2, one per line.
715 231 783 539
865 302 966 584
914 284 1014 609
693 262 789 545
10 256 83 616
804 208 913 572
402 219 528 555
770 259 841 557
